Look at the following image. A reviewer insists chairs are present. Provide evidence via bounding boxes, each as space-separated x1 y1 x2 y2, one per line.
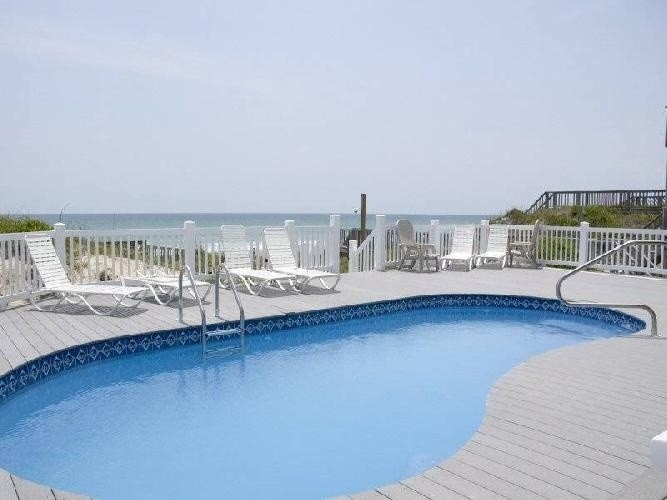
394 218 542 270
23 232 150 316
261 227 341 294
118 267 212 306
218 225 299 297
